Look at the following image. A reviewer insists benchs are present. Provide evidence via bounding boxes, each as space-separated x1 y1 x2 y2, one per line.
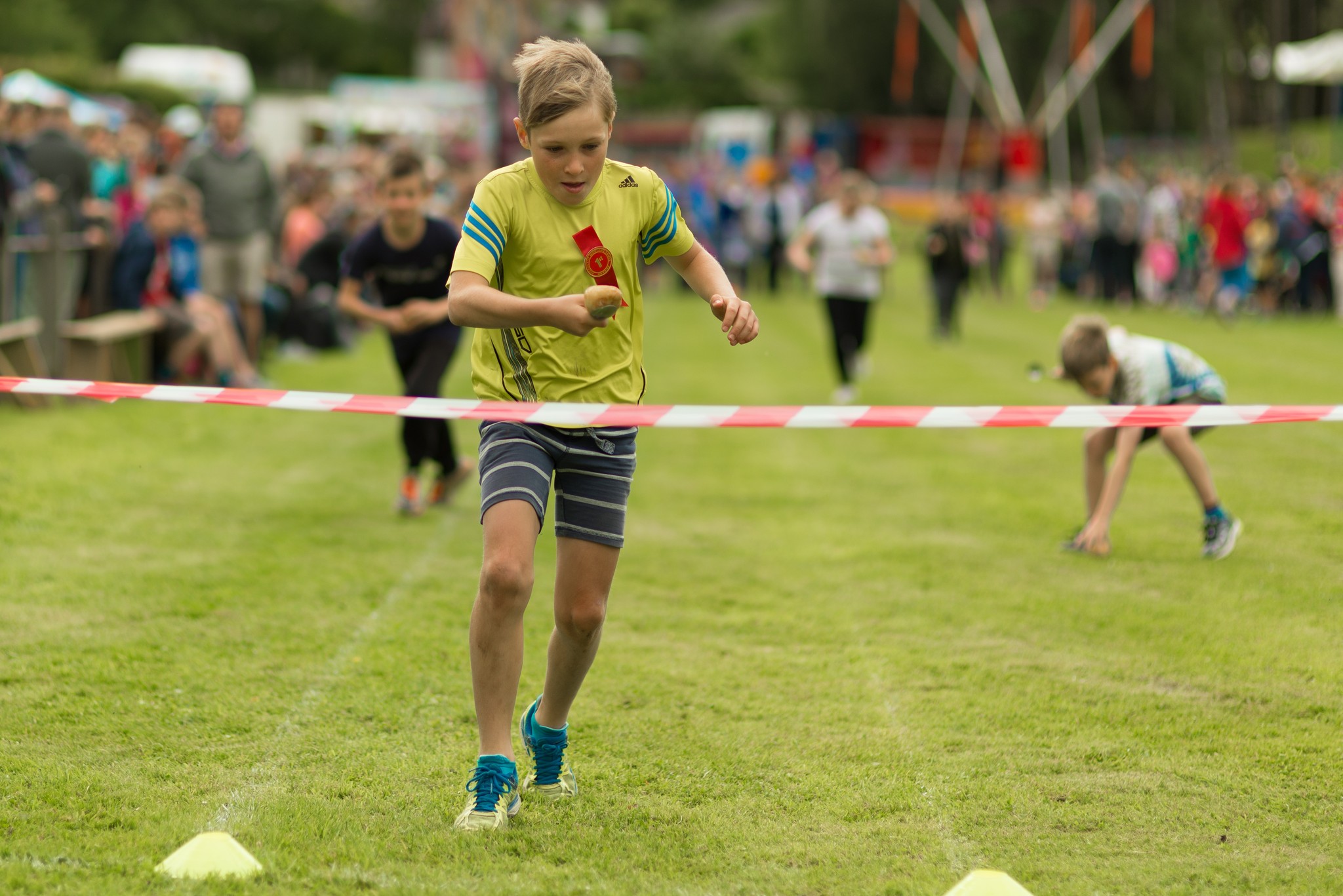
57 303 181 395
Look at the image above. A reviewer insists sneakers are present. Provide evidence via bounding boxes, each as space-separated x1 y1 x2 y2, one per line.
1063 524 1112 554
451 754 521 835
394 456 476 517
1200 511 1245 561
519 693 578 802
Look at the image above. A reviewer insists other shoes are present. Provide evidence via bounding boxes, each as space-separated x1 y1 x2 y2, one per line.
155 364 272 386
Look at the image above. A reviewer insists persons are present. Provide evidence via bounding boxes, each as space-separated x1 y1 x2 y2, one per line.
332 149 479 518
444 33 760 831
782 167 895 403
1046 314 1244 564
919 148 1343 346
1 53 841 406
